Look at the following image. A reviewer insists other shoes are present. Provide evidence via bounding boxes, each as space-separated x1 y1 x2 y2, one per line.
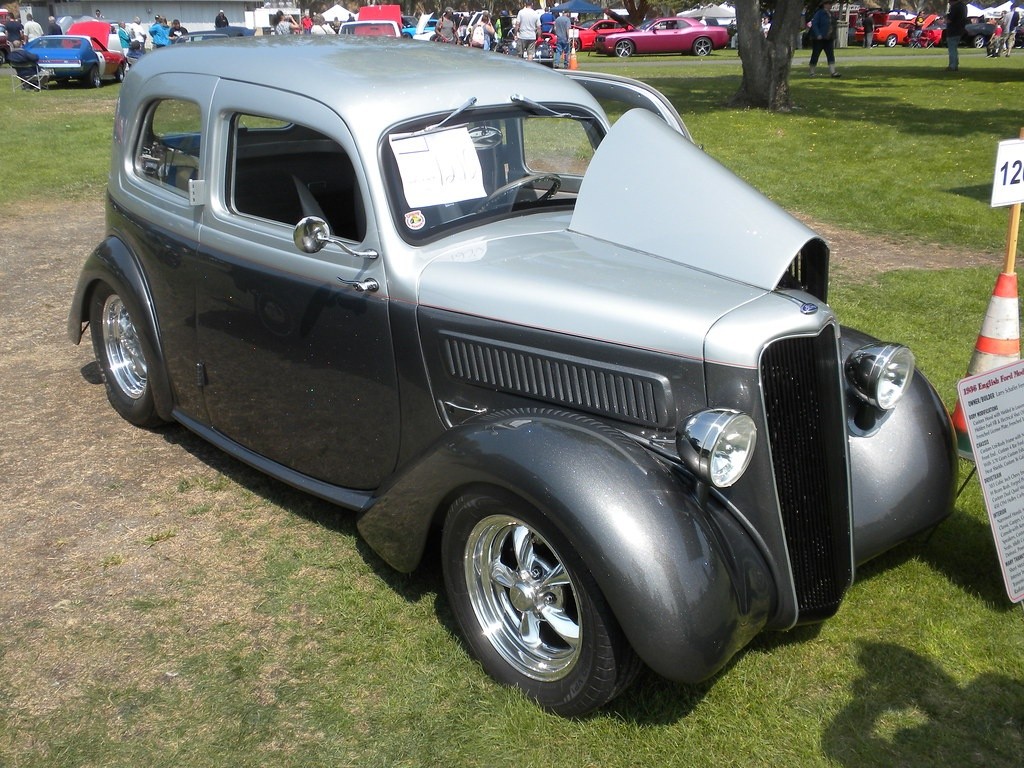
831 72 841 77
809 73 818 78
563 65 567 68
554 64 558 68
1006 53 1010 57
1002 49 1006 53
945 67 957 71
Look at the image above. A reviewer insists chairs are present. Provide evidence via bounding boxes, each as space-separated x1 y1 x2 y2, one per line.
125 56 138 68
7 50 51 94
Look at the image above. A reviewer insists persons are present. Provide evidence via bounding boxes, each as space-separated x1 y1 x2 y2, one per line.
0 10 190 90
863 11 875 49
985 4 1024 58
762 17 771 37
435 0 581 69
806 0 843 78
215 10 228 28
942 0 967 72
915 9 926 30
271 11 355 36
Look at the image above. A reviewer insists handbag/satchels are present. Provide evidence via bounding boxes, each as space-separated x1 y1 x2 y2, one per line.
132 40 140 49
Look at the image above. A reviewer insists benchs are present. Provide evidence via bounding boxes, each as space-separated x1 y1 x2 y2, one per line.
160 139 354 206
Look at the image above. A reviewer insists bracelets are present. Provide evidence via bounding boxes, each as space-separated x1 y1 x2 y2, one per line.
442 35 444 36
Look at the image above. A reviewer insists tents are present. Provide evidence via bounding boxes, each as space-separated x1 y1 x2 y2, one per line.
550 0 604 22
319 5 359 28
676 1 738 25
965 0 1024 23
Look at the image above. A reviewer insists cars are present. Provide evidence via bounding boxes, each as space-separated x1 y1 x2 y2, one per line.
540 19 634 52
855 12 1024 49
338 20 403 39
174 26 257 43
594 18 730 56
0 23 10 66
66 33 959 721
401 16 419 28
0 9 8 25
401 19 438 39
20 22 127 89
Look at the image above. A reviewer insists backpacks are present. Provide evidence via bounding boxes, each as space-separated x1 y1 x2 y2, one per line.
472 23 488 49
1010 12 1019 31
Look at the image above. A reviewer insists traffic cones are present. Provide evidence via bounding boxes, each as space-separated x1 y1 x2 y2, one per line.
949 269 1021 460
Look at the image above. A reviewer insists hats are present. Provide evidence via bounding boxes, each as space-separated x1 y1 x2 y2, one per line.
562 9 570 14
220 10 224 13
155 15 160 19
445 7 454 13
819 0 834 5
